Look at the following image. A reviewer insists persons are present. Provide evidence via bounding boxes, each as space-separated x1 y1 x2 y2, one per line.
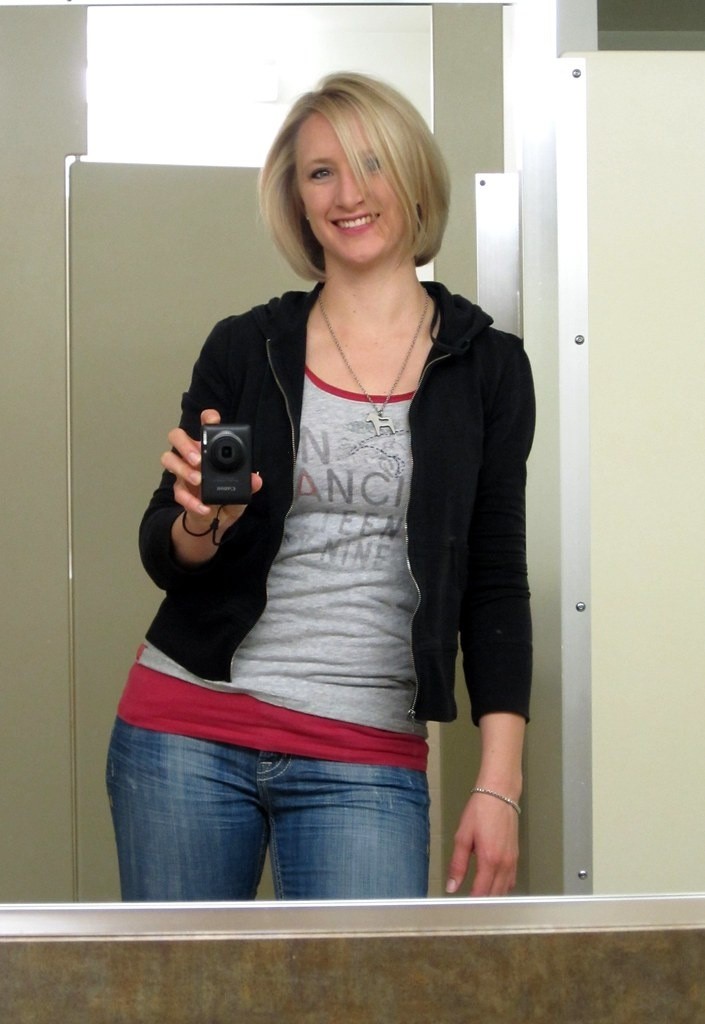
101 71 543 905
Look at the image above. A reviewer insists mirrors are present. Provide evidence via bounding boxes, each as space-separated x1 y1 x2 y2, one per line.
0 0 705 930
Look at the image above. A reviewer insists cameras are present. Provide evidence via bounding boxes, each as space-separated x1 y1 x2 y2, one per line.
200 423 253 505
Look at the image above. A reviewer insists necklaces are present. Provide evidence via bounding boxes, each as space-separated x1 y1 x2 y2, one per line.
316 286 433 436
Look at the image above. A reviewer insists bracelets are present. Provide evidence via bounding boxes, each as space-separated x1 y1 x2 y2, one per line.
471 787 522 814
